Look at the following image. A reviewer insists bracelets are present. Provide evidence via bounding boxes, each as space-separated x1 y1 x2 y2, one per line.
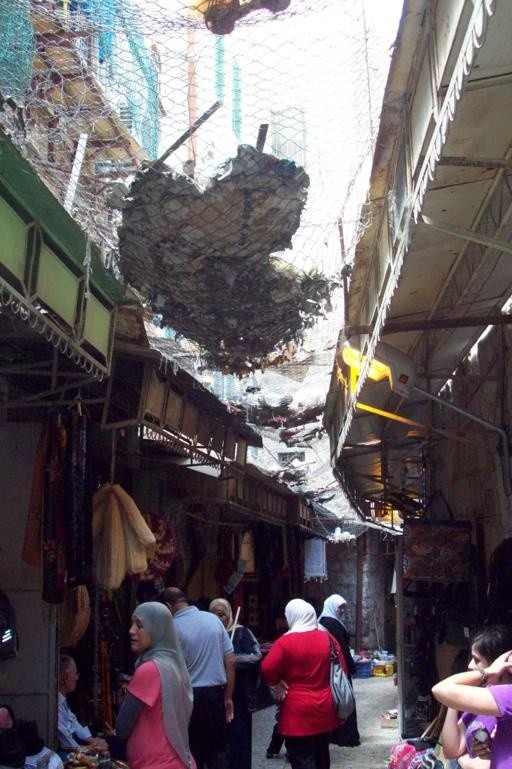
473 667 487 686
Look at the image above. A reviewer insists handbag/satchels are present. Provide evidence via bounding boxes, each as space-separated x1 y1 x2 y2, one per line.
236 626 276 711
328 633 356 721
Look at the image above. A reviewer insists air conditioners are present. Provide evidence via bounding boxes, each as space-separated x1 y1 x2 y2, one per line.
304 537 328 583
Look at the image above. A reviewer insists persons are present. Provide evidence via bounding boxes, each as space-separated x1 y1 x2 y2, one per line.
157 587 234 769
432 650 512 769
112 603 199 769
260 599 350 769
208 598 262 769
57 655 108 758
441 629 512 769
266 702 284 759
318 594 361 747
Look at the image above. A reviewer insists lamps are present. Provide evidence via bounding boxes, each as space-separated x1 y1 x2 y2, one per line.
343 334 508 454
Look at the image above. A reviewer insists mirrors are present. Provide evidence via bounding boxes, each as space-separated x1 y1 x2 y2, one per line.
397 523 470 742
